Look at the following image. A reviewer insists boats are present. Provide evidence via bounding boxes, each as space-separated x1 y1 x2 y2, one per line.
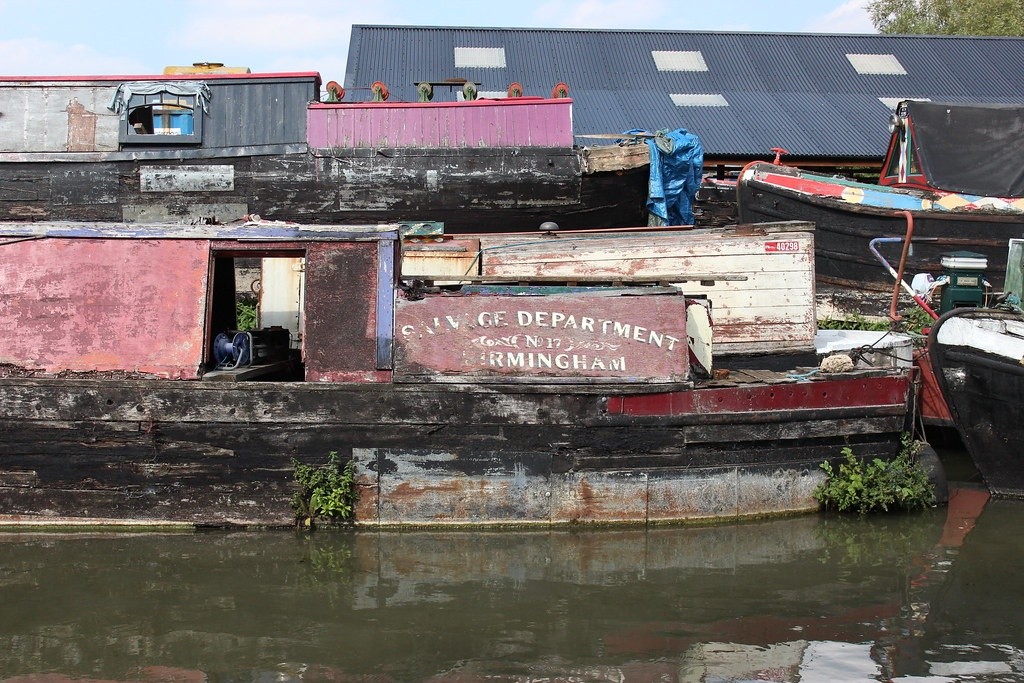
0 137 1024 533
